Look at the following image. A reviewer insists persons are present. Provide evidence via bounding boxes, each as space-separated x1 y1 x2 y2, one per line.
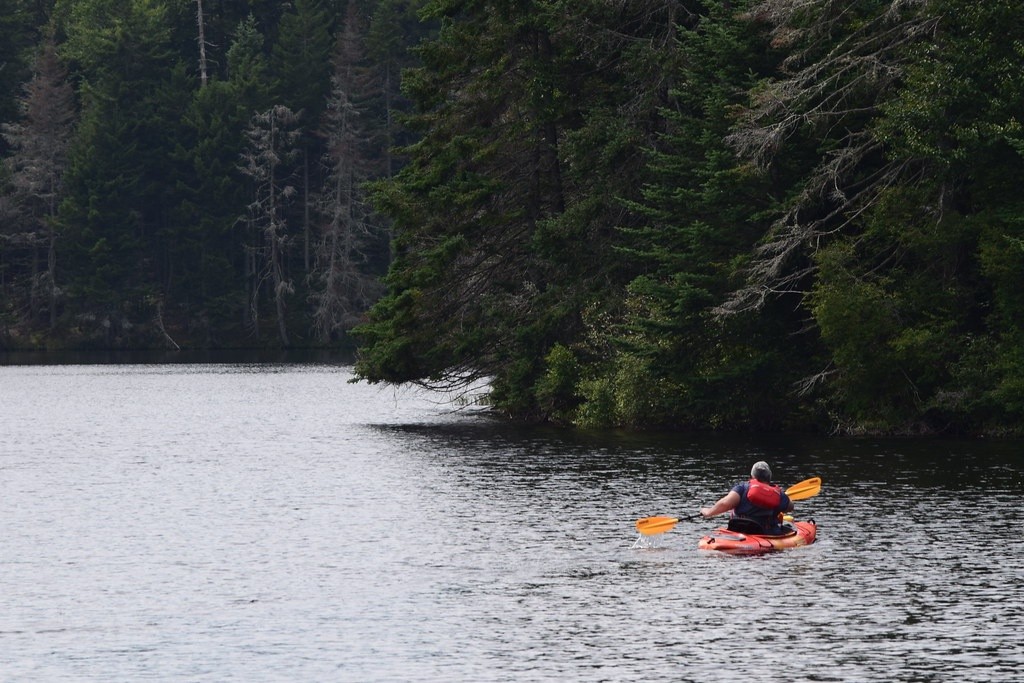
700 461 794 533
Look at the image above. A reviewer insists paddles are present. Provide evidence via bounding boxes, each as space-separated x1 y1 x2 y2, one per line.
633 473 824 536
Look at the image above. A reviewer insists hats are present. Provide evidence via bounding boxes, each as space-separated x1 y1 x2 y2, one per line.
751 461 772 480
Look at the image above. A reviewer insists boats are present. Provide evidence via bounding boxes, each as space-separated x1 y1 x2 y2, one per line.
697 515 819 556
